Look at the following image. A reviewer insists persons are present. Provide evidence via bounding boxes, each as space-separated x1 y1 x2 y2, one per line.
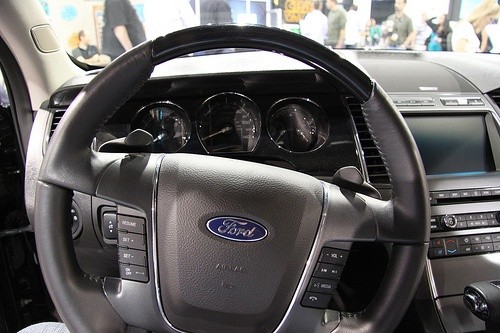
300 0 328 47
424 13 452 51
72 30 100 64
460 0 500 53
344 5 362 48
324 0 347 49
365 0 415 49
100 0 146 62
